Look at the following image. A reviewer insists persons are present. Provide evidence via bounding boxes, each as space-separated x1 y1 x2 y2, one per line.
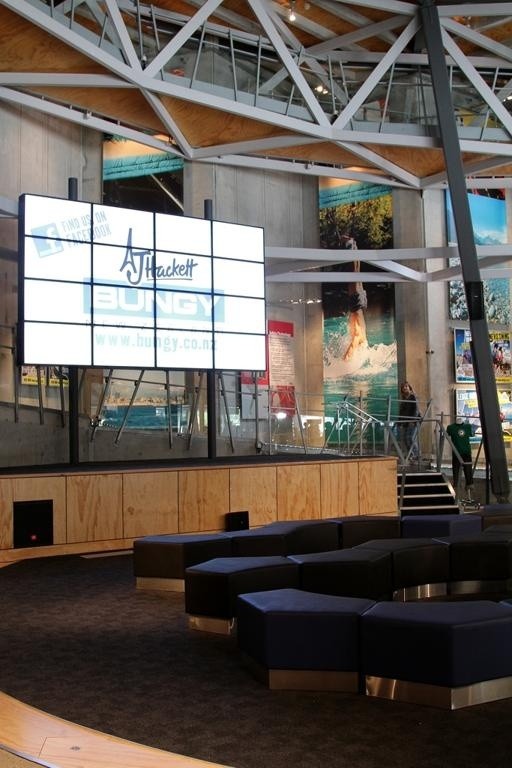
396 381 422 464
333 225 371 364
491 342 498 365
460 346 473 367
444 417 476 500
495 345 506 367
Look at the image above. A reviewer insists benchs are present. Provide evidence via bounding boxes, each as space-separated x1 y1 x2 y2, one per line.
183 533 512 635
132 510 511 594
236 590 512 709
